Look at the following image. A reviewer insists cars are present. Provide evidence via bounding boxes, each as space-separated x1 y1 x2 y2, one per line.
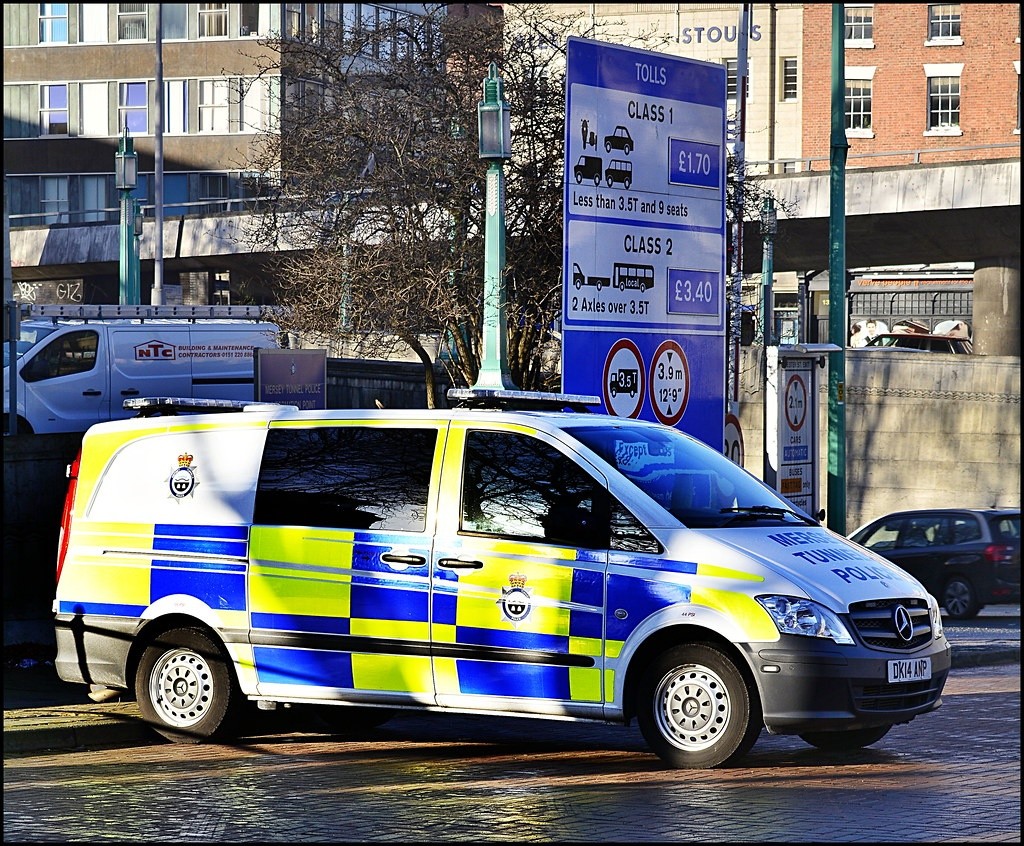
866 332 974 355
846 509 1022 621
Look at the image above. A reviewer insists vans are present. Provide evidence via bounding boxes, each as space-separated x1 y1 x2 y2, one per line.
4 317 282 435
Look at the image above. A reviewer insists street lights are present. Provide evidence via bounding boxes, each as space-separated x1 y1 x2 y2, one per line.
132 196 143 305
115 128 139 305
755 196 778 346
477 62 518 390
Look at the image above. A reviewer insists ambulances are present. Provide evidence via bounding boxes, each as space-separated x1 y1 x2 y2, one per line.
51 389 953 770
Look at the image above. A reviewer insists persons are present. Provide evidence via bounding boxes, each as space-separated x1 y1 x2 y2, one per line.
856 318 886 347
